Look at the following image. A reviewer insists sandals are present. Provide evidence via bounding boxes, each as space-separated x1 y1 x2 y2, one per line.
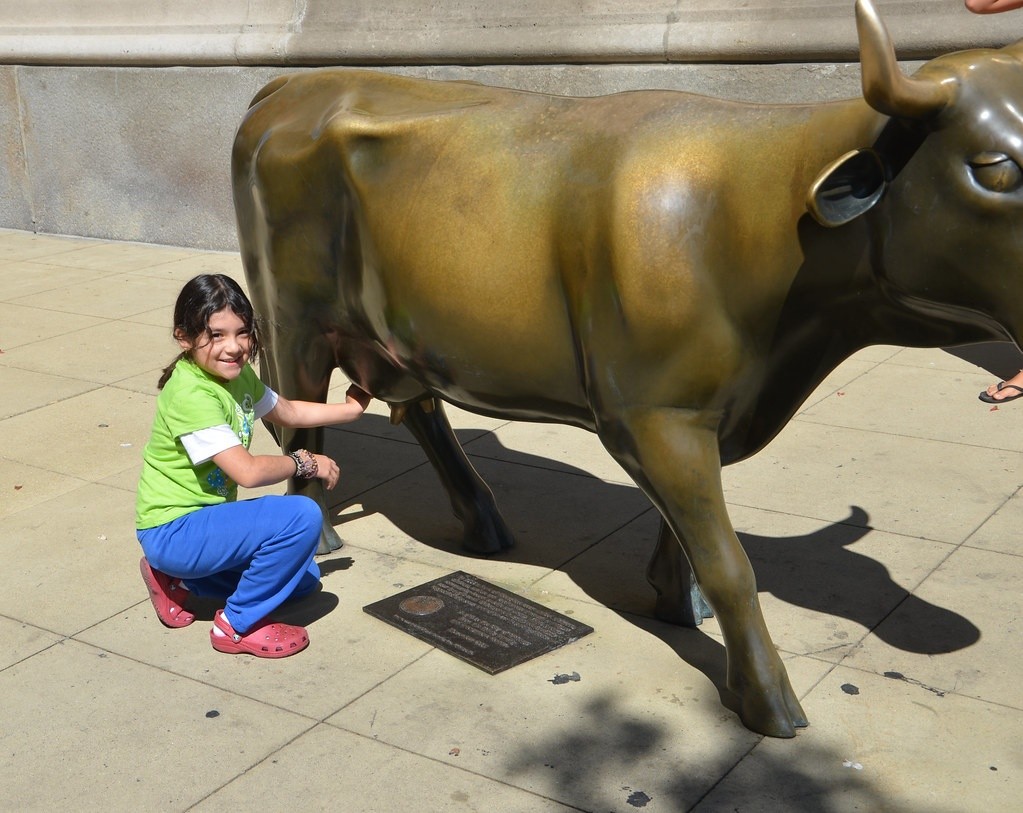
210 606 309 657
140 555 196 628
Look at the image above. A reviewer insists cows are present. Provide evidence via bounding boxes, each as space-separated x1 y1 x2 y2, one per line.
230 0 1023 741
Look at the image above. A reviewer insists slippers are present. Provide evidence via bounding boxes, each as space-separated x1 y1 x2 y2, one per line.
979 380 1023 403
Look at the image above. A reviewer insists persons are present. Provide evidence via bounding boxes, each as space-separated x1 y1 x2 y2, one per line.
135 274 371 660
964 0 1023 403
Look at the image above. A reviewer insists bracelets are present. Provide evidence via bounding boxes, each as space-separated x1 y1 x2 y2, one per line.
287 452 305 478
298 448 318 479
